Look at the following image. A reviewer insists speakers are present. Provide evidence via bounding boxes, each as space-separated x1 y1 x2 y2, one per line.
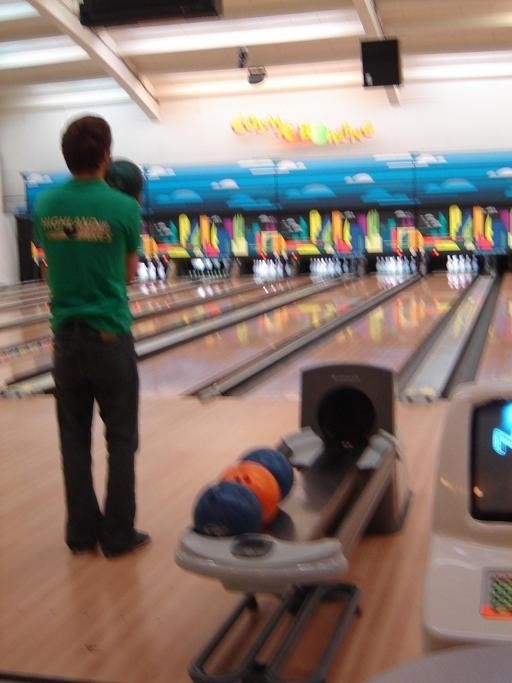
360 40 401 86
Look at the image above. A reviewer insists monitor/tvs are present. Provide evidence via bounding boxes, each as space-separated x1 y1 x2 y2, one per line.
79 1 224 28
469 399 512 524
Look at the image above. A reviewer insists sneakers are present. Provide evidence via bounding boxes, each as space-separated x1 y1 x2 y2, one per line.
99 531 148 558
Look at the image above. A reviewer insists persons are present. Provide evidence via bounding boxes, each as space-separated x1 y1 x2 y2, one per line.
30 112 152 558
